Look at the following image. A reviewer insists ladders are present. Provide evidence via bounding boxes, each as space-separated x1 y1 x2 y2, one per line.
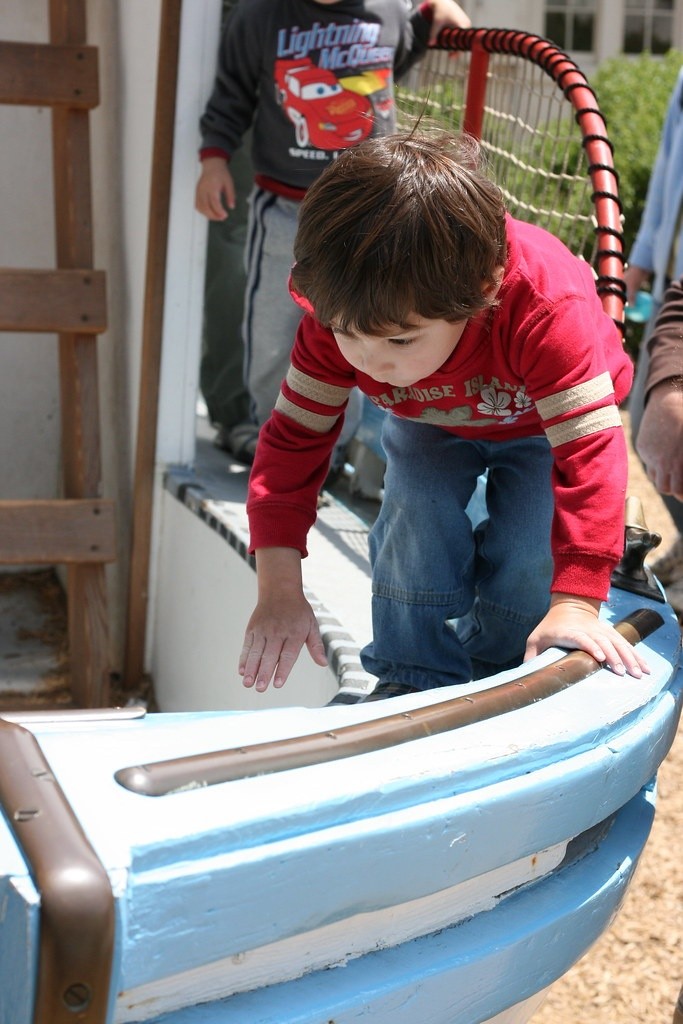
0 0 118 709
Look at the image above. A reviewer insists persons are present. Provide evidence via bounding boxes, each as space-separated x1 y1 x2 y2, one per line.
624 70 683 528
196 0 473 492
238 132 652 704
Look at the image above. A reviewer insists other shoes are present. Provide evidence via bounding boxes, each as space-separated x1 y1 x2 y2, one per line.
214 427 260 465
651 539 683 584
363 684 419 701
